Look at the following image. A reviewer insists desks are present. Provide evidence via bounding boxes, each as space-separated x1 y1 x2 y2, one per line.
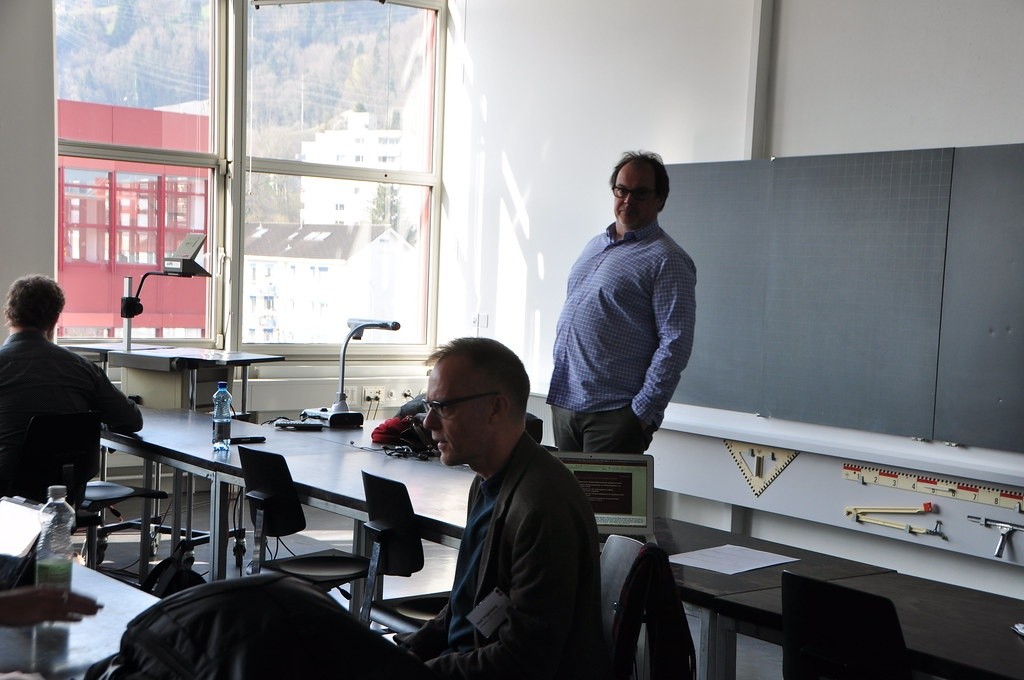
0 562 165 680
95 402 1024 680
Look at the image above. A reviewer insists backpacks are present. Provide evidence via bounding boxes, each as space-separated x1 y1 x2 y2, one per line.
77 572 437 680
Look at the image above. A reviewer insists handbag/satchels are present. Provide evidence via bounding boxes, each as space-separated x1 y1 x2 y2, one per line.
372 413 439 455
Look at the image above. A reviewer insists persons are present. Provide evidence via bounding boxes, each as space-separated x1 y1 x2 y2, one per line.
545 149 698 556
392 337 609 680
0 273 143 510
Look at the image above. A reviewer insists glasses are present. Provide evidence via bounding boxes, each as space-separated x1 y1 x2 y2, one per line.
421 393 501 417
612 183 662 200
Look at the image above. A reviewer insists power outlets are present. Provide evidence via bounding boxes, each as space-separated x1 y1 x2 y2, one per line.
363 387 428 405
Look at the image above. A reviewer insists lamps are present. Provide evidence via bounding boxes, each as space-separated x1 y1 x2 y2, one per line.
298 315 400 431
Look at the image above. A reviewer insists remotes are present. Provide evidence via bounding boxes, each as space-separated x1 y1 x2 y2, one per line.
276 421 323 430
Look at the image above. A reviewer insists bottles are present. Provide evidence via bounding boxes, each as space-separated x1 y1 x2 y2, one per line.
35 484 75 601
211 382 232 452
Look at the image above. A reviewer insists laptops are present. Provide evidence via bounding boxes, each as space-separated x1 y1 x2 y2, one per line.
549 450 657 553
0 495 56 592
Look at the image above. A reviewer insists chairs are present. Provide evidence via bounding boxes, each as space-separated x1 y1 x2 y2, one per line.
782 569 913 680
19 410 104 569
237 445 368 596
357 467 457 642
81 469 168 567
599 535 654 674
